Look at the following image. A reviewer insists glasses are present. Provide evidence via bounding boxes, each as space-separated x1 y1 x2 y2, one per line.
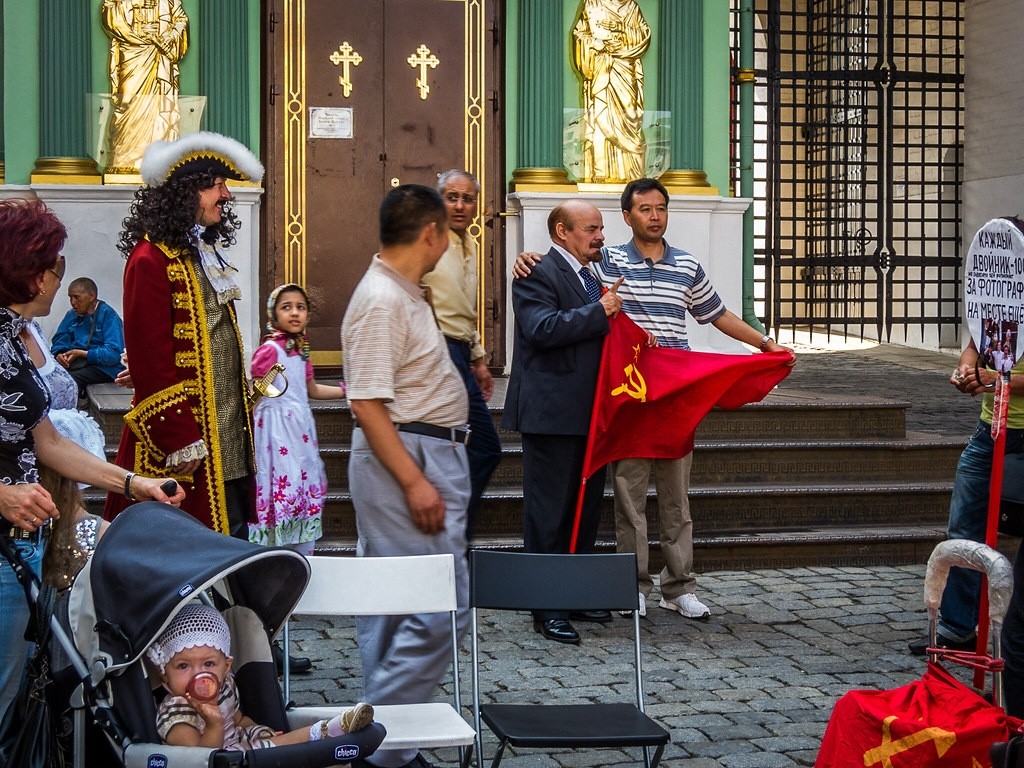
438 192 476 204
46 256 65 282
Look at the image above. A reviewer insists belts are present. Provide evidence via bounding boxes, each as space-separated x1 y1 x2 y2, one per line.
354 418 472 445
444 336 471 348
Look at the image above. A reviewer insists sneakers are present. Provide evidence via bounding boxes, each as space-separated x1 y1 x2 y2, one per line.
618 592 647 617
658 592 711 618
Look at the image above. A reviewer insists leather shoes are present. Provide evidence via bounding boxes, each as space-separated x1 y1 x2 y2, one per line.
270 639 312 674
569 611 612 622
533 616 581 643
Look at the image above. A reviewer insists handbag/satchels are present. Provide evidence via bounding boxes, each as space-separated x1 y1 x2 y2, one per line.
63 357 88 372
0 582 65 768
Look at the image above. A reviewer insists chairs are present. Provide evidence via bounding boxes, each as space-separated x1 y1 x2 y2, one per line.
279 553 477 768
463 549 671 768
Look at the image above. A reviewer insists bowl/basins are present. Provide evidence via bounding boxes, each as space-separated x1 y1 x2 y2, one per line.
186 672 220 703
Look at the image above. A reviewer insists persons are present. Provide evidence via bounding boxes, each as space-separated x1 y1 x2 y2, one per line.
114 347 135 388
909 216 1024 655
146 604 373 757
423 167 504 553
501 199 660 645
339 184 471 768
249 285 344 556
573 0 652 184
1001 539 1024 719
0 192 187 741
50 278 124 406
99 0 191 173
980 320 1017 374
101 132 312 675
512 178 799 621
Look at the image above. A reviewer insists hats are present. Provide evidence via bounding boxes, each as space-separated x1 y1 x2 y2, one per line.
140 129 266 189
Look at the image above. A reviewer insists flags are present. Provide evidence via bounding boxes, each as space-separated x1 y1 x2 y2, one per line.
587 289 793 480
813 660 1010 768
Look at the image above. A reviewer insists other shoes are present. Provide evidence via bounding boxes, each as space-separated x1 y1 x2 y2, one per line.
321 701 374 739
908 630 977 654
350 751 439 768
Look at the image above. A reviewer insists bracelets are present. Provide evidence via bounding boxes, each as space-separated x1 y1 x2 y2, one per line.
760 336 774 352
124 472 139 502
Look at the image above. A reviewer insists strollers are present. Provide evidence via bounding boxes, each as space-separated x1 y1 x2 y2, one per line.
0 480 387 767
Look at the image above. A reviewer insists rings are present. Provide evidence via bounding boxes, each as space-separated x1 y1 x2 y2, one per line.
31 517 37 523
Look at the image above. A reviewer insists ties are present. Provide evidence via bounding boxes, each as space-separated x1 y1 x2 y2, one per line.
578 267 601 304
